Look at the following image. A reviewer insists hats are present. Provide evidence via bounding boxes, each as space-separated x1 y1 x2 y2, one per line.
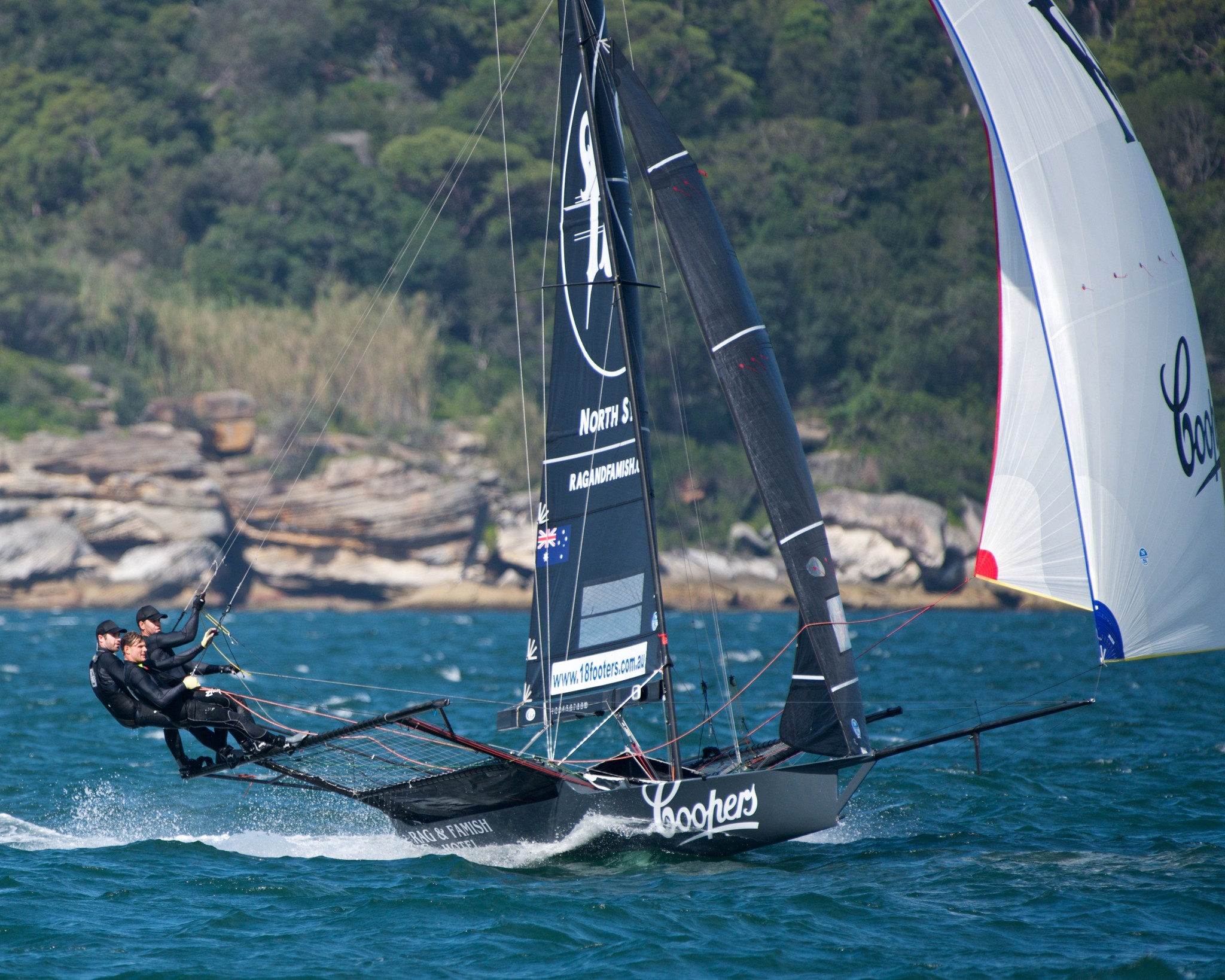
136 605 168 625
96 619 127 639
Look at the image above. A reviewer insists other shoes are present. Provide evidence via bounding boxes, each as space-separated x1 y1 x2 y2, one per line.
219 745 244 769
179 759 198 778
239 739 273 760
283 730 309 756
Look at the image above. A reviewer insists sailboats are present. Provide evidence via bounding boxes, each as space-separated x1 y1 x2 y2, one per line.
174 1 1225 872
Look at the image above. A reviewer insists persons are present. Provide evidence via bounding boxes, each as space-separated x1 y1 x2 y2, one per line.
89 620 244 780
121 628 311 757
136 592 274 765
